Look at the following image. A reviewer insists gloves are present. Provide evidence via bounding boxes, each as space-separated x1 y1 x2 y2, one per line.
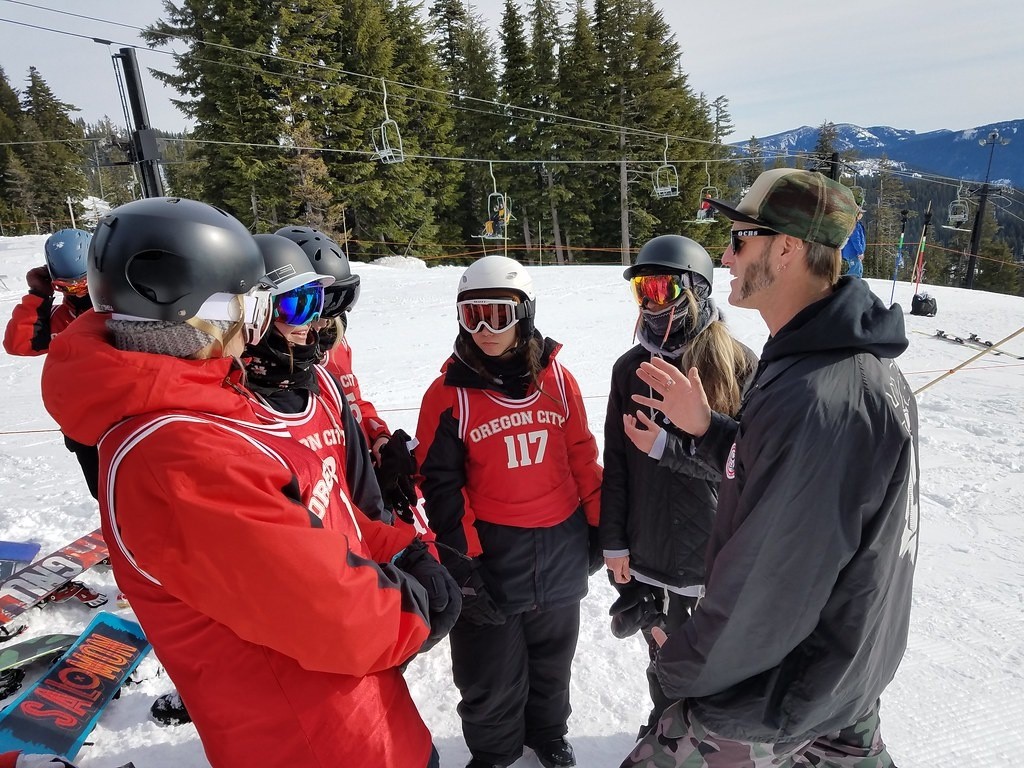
436 543 506 628
586 524 605 575
398 536 463 652
25 264 55 298
603 569 666 639
373 426 419 523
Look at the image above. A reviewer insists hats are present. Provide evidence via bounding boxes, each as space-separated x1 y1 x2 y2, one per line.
858 207 866 213
702 168 861 250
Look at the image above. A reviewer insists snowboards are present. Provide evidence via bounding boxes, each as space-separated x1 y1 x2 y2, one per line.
0 632 80 675
1 611 155 767
1 525 109 629
1 540 40 565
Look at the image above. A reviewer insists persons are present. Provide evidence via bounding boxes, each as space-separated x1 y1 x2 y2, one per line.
273 224 418 531
620 167 920 768
485 203 511 238
2 229 98 500
414 253 602 768
843 204 869 278
239 233 392 526
607 233 758 664
40 197 462 767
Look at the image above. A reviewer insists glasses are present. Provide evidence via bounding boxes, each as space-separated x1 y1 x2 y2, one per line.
242 284 274 346
269 280 324 326
457 297 536 334
321 273 360 317
51 278 89 296
628 269 682 306
730 228 780 255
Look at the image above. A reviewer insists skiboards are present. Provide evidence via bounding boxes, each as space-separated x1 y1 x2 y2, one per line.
682 218 719 225
47 577 108 610
914 321 1023 364
471 232 511 241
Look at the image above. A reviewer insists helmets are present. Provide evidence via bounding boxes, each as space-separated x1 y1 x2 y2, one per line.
250 232 336 300
273 226 350 281
457 255 536 341
85 194 264 324
623 234 714 297
44 229 93 280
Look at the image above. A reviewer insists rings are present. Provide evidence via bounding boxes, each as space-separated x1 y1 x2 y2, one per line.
664 379 675 390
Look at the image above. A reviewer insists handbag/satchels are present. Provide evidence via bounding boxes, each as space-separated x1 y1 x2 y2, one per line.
910 293 938 316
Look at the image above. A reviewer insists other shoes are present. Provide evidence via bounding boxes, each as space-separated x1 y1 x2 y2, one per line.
522 732 576 768
464 743 523 768
149 691 191 726
